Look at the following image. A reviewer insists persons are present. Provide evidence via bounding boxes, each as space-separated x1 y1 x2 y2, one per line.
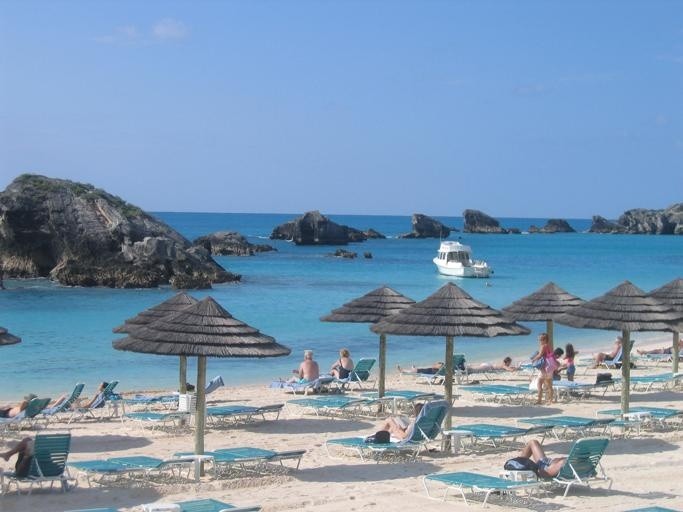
382 403 423 439
0 437 51 477
71 382 108 408
521 439 594 478
0 394 36 418
593 337 621 367
46 395 68 408
397 334 578 405
285 350 319 384
328 349 354 379
637 341 683 355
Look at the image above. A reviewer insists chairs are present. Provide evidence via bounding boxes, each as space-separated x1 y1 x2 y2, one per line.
0 337 309 512
267 339 683 512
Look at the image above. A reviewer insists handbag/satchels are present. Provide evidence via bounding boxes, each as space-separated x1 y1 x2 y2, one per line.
365 431 390 444
504 457 538 470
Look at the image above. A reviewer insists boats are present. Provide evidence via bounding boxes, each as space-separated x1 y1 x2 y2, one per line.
433 240 490 277
